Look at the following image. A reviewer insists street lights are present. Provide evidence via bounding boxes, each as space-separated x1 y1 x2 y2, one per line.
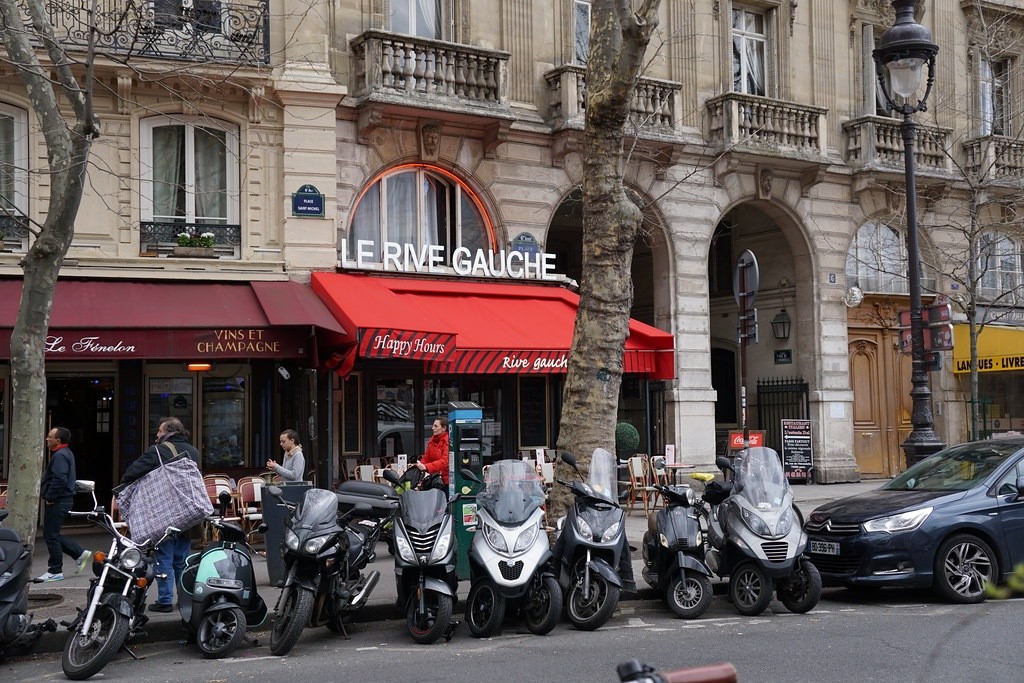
872 1 940 488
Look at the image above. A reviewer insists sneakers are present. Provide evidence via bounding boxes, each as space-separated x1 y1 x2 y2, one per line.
34 572 64 583
74 550 92 576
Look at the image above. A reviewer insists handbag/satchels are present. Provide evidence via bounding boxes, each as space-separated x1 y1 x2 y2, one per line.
422 474 445 491
111 441 215 551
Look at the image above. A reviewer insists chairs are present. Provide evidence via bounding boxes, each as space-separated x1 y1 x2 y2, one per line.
107 461 419 551
627 456 689 520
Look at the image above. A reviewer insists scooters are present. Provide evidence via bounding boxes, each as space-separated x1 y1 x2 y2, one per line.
267 480 400 656
176 487 269 659
641 459 716 620
545 448 639 634
0 509 45 659
382 469 463 644
459 459 564 638
59 480 183 680
696 446 823 617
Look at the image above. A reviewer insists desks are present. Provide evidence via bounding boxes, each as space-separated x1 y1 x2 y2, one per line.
663 463 696 487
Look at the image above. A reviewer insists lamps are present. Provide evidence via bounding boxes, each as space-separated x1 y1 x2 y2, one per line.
771 309 791 339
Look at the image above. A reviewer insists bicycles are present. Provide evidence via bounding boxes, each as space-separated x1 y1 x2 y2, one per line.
687 472 804 556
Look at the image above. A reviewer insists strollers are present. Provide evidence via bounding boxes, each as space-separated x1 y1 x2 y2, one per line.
381 464 425 555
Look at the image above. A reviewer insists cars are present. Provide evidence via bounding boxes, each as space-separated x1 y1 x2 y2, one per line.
802 436 1024 605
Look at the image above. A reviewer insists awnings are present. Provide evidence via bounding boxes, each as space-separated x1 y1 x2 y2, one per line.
0 281 346 367
953 324 1024 373
311 273 675 490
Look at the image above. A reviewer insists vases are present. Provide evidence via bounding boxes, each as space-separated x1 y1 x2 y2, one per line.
173 246 214 257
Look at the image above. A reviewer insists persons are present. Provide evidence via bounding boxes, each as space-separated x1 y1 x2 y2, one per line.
417 417 449 484
37 427 92 582
121 417 200 613
266 430 305 481
213 436 241 467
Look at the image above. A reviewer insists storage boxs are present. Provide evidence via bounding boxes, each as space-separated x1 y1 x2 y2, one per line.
338 479 399 518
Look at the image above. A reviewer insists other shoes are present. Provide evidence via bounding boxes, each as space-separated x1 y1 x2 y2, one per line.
148 601 173 613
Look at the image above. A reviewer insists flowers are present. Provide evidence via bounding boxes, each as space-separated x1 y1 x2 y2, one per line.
177 232 215 248
